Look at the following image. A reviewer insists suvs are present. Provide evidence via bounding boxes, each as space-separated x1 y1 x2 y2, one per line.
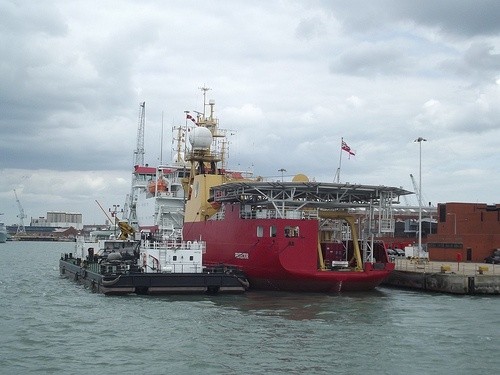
490 248 500 256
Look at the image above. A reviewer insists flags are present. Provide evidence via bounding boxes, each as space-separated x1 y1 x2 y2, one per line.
342 141 355 156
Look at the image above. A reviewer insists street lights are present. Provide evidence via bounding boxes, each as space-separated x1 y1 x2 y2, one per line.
277 168 287 182
413 136 428 266
448 213 457 236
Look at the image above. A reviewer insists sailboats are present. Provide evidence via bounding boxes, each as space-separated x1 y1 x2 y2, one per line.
149 110 168 193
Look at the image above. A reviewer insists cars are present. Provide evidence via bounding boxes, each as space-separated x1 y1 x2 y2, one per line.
387 248 405 256
483 255 500 264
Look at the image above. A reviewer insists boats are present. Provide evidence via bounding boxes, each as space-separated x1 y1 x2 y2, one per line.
58 204 250 298
0 212 9 244
130 84 418 292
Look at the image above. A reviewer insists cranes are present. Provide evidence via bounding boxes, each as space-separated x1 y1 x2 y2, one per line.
13 188 28 237
126 101 146 220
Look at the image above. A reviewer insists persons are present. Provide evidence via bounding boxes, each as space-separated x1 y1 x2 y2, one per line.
148 233 154 246
94 254 98 264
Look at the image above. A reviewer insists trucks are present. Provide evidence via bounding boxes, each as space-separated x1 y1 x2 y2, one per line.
404 247 430 262
